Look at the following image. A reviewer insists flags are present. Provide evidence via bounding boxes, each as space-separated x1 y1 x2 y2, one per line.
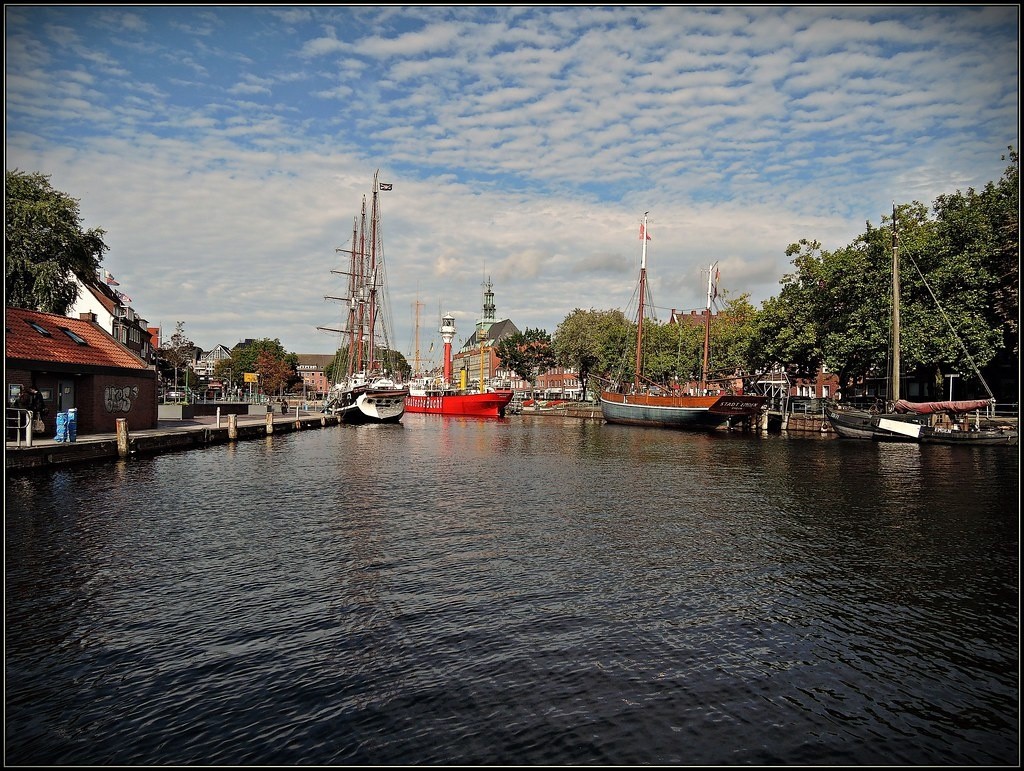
640 225 651 240
380 183 392 190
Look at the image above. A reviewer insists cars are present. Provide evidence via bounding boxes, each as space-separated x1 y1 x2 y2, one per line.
165 385 198 403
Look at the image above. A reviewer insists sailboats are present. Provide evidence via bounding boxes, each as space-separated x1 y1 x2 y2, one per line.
820 199 1022 447
600 209 768 429
317 168 411 425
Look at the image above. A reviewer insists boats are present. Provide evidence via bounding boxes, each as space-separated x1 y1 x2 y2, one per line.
403 300 515 418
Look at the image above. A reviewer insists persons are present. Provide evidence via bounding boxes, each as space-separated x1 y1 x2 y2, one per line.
29 386 45 439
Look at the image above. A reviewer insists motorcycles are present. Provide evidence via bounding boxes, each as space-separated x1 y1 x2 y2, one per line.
280 398 288 415
